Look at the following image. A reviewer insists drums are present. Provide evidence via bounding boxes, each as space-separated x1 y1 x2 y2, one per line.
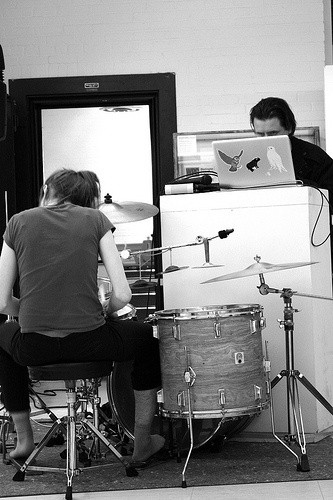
155 304 271 420
97 277 136 320
107 314 255 450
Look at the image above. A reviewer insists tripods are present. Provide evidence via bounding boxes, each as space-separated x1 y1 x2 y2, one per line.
203 274 333 473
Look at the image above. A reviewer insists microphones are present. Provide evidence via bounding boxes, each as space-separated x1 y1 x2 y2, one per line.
169 175 213 184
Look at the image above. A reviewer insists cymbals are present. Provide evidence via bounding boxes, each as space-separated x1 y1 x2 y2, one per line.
155 265 190 275
191 262 224 268
96 201 160 224
200 262 319 284
129 280 157 289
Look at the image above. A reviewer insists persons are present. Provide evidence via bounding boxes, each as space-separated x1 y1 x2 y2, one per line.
250 97 333 216
0 169 164 464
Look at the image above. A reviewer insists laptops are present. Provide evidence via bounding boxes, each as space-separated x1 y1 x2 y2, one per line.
212 135 296 189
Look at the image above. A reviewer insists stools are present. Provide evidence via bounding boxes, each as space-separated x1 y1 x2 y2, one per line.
12 359 139 500
